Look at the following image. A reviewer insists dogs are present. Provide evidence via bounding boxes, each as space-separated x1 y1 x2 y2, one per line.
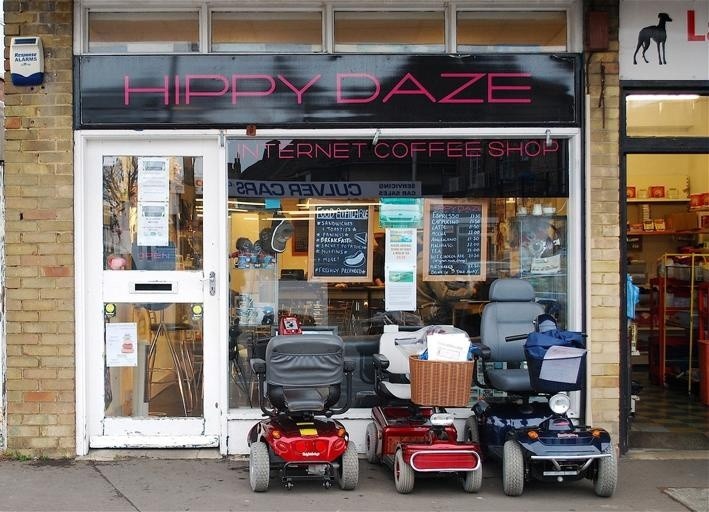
631 11 673 65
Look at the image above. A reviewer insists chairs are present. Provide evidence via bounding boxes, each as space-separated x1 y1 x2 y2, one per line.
143 304 197 417
372 323 424 405
473 278 555 414
250 335 354 424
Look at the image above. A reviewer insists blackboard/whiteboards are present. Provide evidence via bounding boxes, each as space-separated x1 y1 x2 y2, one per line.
307 198 374 283
422 197 488 283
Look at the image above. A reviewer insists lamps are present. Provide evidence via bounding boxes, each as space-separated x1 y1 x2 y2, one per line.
585 9 610 54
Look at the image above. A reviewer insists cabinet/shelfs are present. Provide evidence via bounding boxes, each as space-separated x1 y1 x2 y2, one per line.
628 199 709 383
